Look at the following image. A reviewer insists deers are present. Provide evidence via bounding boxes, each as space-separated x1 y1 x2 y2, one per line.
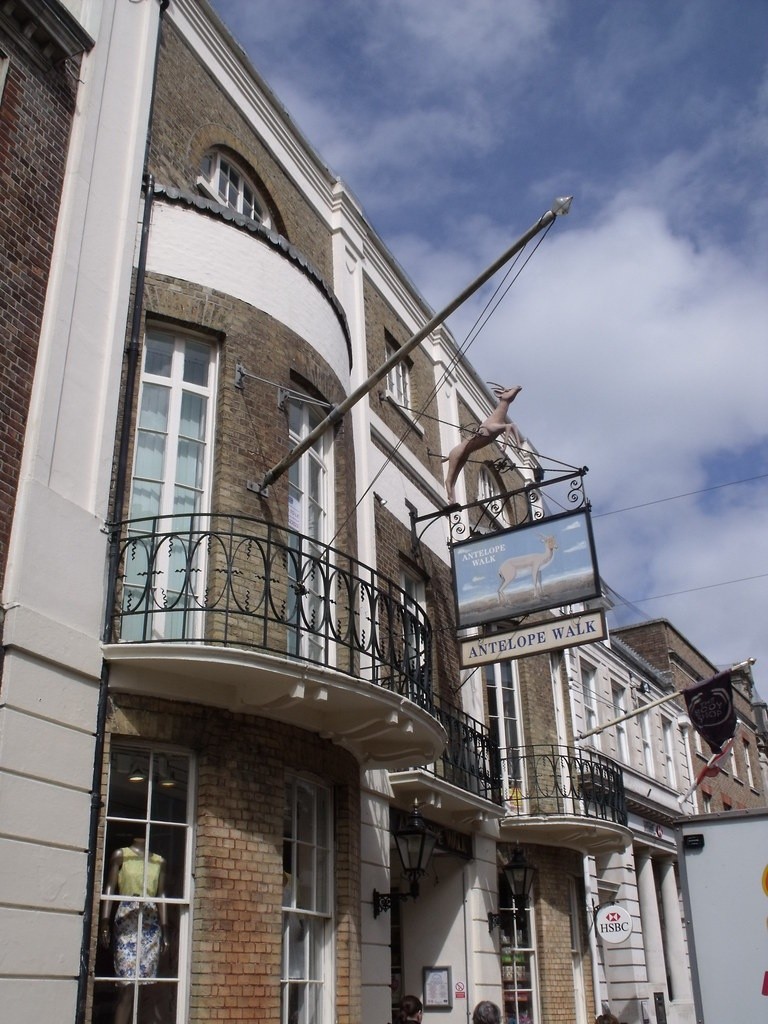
444 379 525 503
496 531 558 604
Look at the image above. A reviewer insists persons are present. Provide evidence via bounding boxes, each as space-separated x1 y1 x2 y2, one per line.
388 994 423 1024
282 871 307 979
595 1014 620 1024
101 836 170 1024
472 1000 501 1024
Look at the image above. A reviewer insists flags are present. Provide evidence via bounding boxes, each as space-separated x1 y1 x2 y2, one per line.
680 668 741 793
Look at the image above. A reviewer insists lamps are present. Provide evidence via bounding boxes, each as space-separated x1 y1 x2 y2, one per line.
371 798 440 919
374 491 387 508
636 682 651 694
488 845 537 934
